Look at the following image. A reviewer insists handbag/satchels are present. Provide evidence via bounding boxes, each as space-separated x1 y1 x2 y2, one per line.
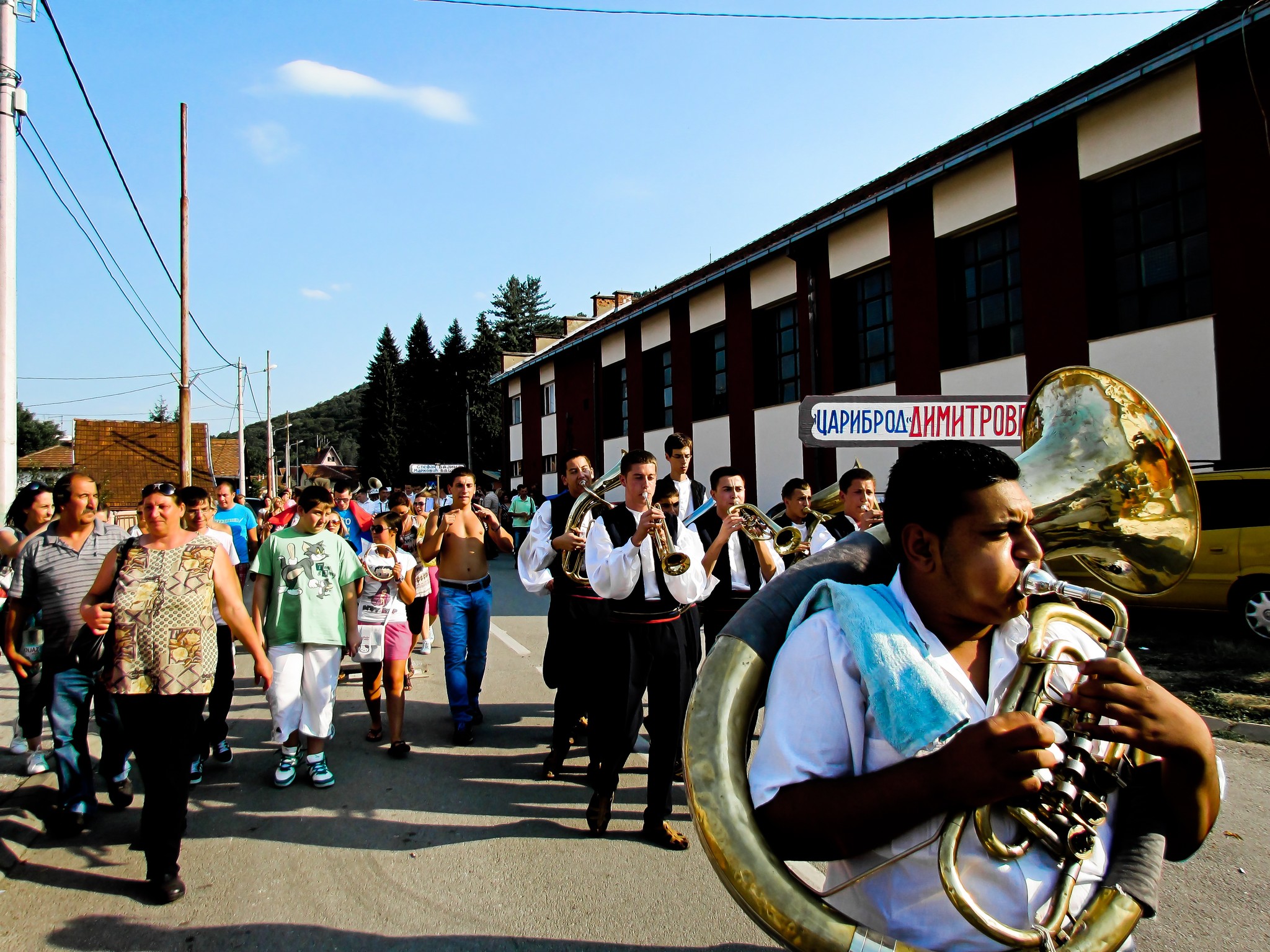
0 557 15 612
351 625 385 662
69 537 136 678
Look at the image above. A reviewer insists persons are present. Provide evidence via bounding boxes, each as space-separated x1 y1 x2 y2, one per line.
810 468 884 555
774 478 825 569
0 433 707 905
689 466 785 655
250 485 368 788
747 443 1225 952
213 481 258 656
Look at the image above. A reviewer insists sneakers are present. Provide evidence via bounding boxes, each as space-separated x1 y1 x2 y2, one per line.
9 716 29 753
109 777 134 807
152 874 186 901
420 624 435 654
190 754 203 783
213 739 233 763
27 751 49 774
274 744 336 788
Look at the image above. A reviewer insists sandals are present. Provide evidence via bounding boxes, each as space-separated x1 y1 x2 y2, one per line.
404 656 414 691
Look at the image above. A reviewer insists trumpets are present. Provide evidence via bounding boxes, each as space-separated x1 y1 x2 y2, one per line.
727 502 802 555
639 491 693 577
858 499 885 526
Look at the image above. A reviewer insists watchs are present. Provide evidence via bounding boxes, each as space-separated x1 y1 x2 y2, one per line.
394 574 404 584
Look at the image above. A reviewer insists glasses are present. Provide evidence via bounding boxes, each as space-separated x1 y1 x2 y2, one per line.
669 455 692 459
142 484 176 497
185 509 210 516
413 502 425 507
326 520 341 527
29 482 48 490
335 498 349 503
370 525 394 533
136 510 143 515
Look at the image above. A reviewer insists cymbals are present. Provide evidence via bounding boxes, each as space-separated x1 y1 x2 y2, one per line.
362 543 398 584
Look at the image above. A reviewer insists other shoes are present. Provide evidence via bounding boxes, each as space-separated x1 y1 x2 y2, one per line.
545 716 690 850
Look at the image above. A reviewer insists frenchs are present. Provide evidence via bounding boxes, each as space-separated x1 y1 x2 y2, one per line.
772 451 883 568
559 446 629 592
679 362 1204 952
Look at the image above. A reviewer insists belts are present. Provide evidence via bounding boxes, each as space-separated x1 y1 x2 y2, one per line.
439 573 491 592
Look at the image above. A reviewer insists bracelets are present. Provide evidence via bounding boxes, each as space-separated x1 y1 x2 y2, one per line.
490 522 501 531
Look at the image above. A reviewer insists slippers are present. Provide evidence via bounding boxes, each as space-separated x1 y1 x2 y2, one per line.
366 727 410 759
